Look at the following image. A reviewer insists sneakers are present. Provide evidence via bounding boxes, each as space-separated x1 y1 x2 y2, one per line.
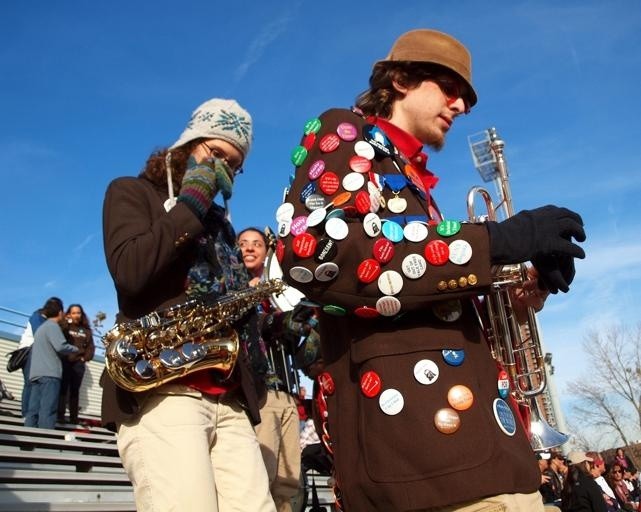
56 416 79 424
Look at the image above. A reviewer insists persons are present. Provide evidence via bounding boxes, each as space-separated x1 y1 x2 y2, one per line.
294 387 307 435
276 29 586 512
19 298 62 420
55 304 95 424
235 228 319 512
100 97 278 512
24 302 79 429
534 447 641 512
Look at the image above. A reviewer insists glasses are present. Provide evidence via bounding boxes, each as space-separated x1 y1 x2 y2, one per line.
612 470 623 474
411 67 473 114
200 138 244 176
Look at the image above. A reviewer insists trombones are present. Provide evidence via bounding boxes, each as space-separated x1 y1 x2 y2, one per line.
467 127 569 452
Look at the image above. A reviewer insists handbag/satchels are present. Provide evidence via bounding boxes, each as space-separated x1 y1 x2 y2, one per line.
4 344 32 372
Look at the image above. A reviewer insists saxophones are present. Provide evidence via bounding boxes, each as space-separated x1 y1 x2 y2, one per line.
101 277 289 392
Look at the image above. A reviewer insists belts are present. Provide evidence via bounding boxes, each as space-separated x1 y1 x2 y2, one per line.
197 387 250 412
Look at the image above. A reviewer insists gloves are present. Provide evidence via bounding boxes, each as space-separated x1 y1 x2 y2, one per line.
484 204 587 294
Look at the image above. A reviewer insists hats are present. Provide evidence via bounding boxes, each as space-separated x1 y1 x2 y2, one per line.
566 450 594 466
535 451 551 460
372 30 478 108
167 97 253 160
